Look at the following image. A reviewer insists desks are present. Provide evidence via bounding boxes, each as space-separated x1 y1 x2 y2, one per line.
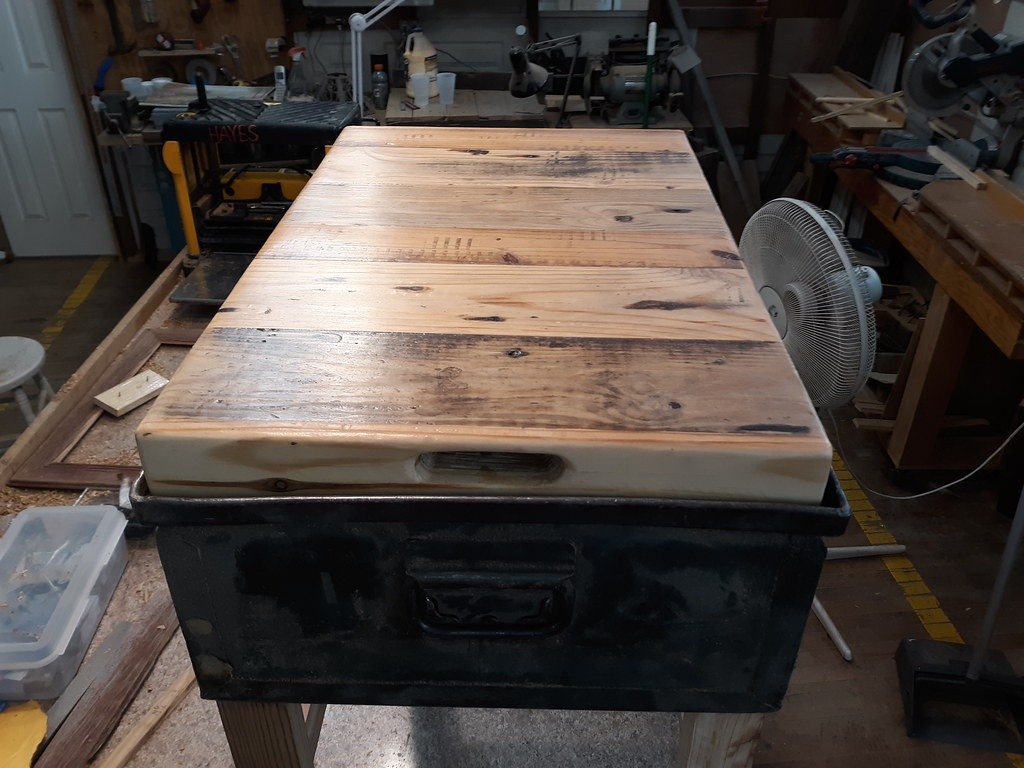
133 126 852 768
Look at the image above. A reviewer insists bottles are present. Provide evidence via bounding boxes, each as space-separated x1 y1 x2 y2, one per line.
371 64 387 109
403 27 443 99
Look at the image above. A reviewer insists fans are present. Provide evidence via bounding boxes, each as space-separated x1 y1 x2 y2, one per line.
740 197 882 407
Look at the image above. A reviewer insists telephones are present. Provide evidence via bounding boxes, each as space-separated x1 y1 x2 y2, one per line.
272 65 286 102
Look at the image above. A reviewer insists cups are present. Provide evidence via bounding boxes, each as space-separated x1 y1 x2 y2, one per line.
436 72 456 106
410 74 430 106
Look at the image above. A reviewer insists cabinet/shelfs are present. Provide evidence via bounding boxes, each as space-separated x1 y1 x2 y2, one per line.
785 67 1024 473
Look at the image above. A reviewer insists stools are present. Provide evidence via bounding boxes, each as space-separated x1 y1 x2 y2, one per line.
1 336 55 428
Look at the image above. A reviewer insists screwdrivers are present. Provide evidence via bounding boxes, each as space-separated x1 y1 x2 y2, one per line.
39 571 73 619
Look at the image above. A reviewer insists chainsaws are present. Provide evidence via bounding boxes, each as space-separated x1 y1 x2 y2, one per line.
808 0 1024 190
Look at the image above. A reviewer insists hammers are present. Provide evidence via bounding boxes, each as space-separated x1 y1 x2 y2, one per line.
104 0 137 57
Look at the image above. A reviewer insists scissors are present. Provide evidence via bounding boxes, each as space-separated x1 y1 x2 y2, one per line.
221 33 243 79
